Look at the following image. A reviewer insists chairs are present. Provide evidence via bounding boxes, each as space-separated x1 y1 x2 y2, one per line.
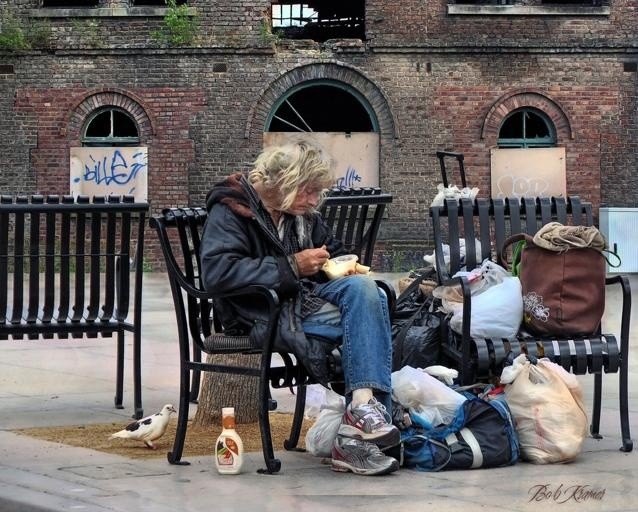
429 195 633 452
148 204 335 474
0 194 149 421
320 186 393 268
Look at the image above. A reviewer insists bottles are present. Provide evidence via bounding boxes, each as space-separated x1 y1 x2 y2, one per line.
215 407 244 475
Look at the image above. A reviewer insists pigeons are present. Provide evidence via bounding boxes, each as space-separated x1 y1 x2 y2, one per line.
108 404 177 450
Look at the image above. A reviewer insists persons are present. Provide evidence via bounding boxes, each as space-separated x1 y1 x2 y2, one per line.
199 141 400 477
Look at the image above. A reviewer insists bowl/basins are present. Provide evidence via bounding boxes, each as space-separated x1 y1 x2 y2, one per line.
320 254 358 280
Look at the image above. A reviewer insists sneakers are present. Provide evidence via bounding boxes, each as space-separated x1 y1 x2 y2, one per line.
332 436 400 475
336 397 400 448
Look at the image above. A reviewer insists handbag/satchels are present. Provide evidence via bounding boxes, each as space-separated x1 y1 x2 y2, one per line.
435 258 524 339
500 222 621 336
392 383 520 473
390 270 452 372
